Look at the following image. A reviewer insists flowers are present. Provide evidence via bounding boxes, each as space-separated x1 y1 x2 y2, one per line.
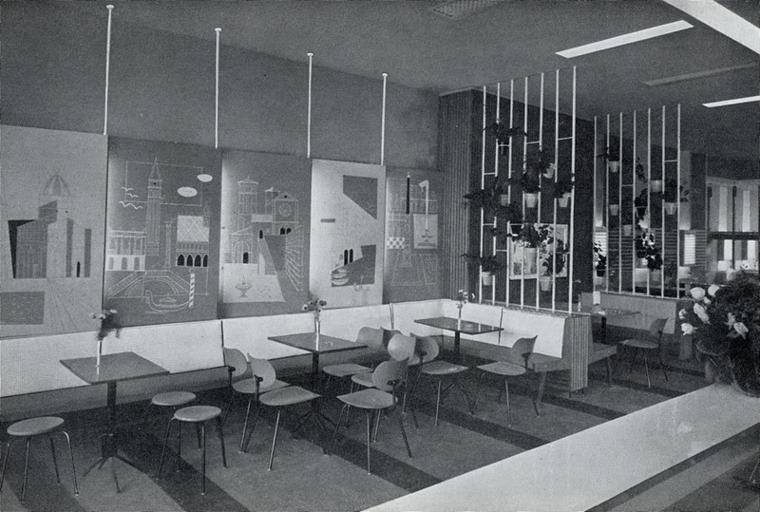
450 290 468 308
675 259 760 398
302 296 328 317
90 309 121 340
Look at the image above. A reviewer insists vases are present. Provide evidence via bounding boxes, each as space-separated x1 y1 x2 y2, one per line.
458 309 461 318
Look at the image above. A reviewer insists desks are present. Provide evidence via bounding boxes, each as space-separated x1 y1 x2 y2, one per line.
579 307 640 343
